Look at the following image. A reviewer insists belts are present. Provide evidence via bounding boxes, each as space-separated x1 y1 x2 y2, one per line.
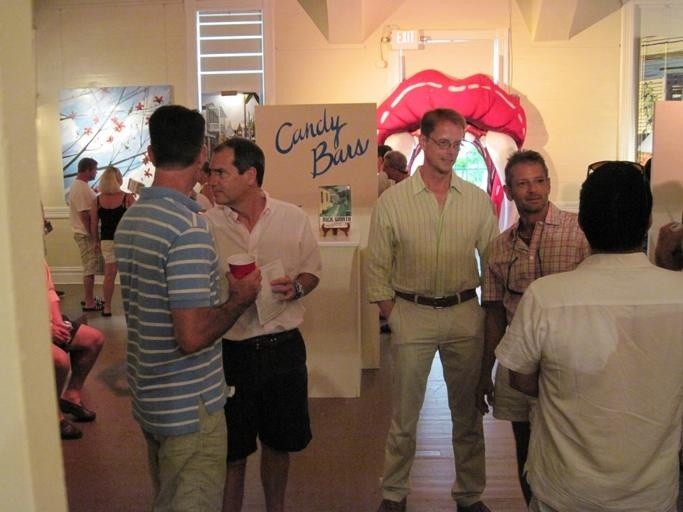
225 330 299 348
396 289 478 308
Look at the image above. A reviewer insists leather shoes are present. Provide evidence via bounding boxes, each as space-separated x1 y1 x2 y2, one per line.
455 501 491 512
377 497 407 511
60 398 96 421
60 419 83 440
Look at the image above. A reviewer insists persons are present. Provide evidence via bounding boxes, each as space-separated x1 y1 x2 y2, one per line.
113 105 261 512
91 165 137 317
197 162 215 212
379 150 409 334
204 138 321 512
377 145 395 319
475 149 592 508
493 162 683 512
69 158 105 312
44 259 106 440
366 109 500 512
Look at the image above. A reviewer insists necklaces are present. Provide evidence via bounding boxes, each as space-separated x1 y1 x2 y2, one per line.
518 225 531 239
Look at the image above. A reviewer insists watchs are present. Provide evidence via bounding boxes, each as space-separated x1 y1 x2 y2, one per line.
293 279 305 300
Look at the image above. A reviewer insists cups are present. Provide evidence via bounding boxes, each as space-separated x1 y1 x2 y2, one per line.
227 254 255 278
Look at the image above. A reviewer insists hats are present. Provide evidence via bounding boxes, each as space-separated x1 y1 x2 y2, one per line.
386 148 410 177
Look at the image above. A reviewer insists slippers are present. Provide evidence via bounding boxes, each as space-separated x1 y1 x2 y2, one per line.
101 309 112 317
81 295 104 304
83 304 104 312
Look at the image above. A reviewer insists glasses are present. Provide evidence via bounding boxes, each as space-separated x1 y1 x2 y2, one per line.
586 160 647 197
424 131 464 152
504 250 545 296
